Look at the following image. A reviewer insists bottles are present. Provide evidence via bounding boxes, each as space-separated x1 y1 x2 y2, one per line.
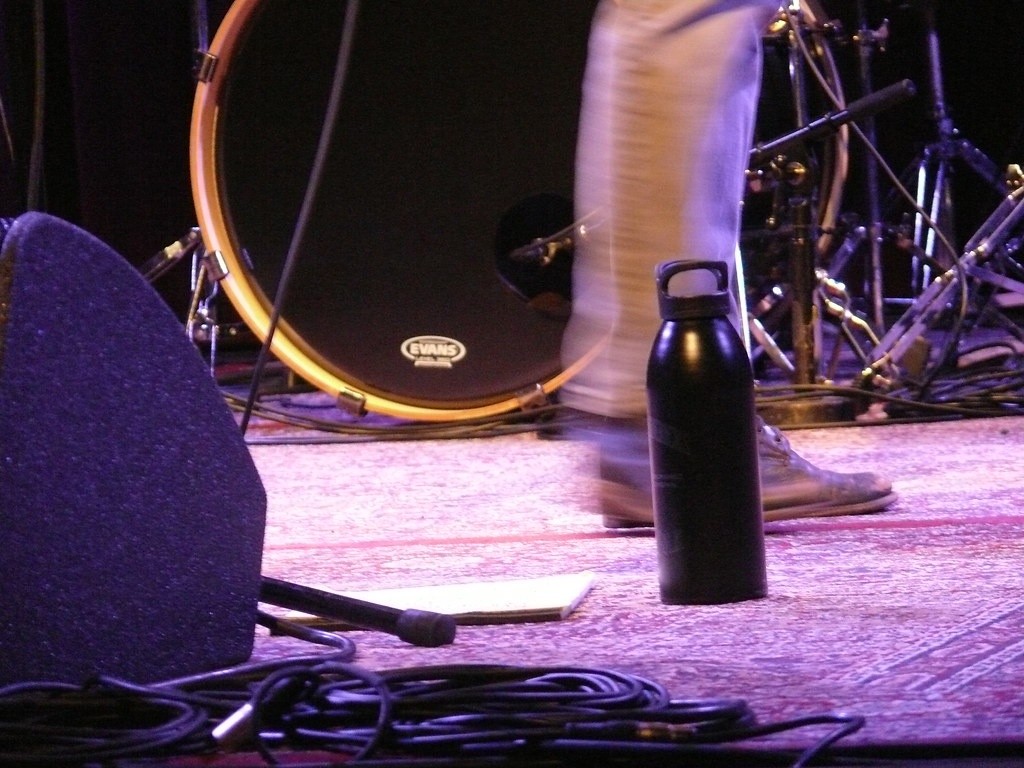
645 258 769 605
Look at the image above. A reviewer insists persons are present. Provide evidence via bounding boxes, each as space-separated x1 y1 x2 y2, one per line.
564 0 898 531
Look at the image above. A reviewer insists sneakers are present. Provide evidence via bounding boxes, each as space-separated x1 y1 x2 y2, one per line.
559 409 898 529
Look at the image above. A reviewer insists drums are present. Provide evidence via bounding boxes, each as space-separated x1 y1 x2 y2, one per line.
194 0 850 431
